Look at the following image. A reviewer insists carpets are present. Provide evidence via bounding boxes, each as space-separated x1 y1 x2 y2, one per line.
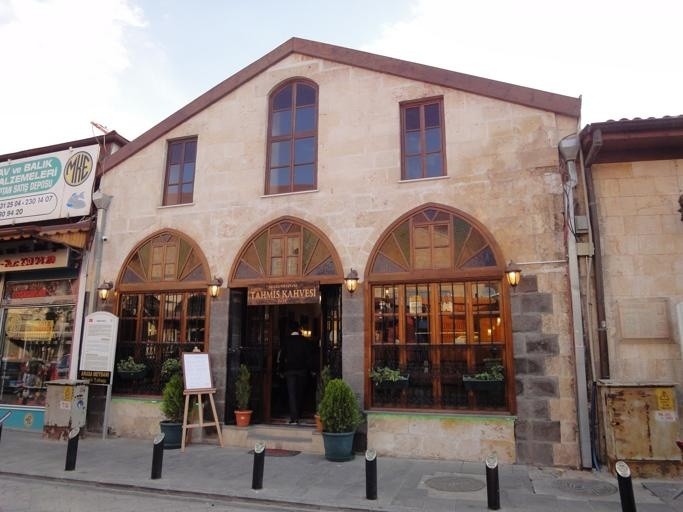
246 447 301 458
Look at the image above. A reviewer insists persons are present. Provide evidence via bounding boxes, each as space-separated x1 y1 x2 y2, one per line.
280 321 312 425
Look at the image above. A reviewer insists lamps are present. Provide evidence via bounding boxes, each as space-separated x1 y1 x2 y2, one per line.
503 259 522 295
342 267 361 300
205 275 223 303
95 280 114 304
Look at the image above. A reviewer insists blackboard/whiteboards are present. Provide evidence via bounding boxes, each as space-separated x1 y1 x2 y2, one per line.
182 352 214 389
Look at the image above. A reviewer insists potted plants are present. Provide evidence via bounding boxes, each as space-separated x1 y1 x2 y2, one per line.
311 363 411 463
113 354 194 450
461 363 506 393
232 362 254 426
